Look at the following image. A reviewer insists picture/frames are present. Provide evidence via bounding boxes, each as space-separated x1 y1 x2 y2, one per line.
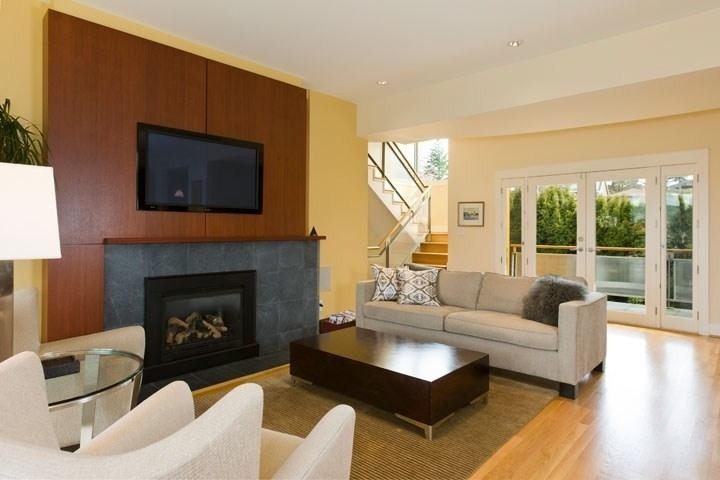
457 201 484 228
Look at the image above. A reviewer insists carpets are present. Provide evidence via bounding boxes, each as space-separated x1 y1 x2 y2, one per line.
194 369 560 480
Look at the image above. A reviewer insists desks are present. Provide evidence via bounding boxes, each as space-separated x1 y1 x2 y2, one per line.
35 349 146 448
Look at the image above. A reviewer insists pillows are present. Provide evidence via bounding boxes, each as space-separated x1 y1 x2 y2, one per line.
372 264 407 302
522 275 591 326
399 267 441 307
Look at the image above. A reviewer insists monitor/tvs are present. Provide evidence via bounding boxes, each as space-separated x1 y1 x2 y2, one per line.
135 120 265 214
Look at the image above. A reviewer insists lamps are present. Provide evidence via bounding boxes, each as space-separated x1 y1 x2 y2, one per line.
2 163 64 260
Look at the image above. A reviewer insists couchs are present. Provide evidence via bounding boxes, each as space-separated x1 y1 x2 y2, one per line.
354 272 609 399
37 325 149 359
0 351 354 480
